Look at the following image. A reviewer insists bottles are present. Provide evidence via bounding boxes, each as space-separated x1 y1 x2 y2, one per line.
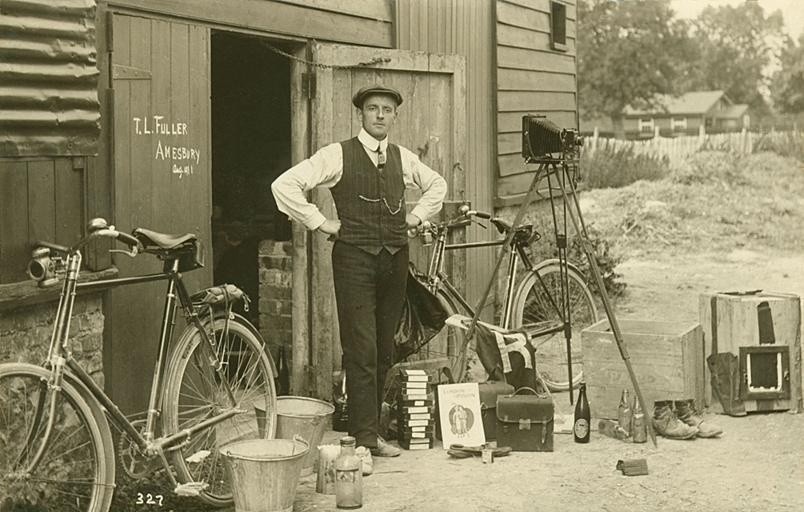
332 435 363 509
617 388 632 436
597 419 629 439
631 395 648 443
573 381 590 443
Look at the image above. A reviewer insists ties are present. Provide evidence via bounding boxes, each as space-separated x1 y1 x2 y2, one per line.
377 147 386 172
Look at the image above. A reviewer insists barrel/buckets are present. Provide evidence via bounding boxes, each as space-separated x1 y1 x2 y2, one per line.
253 395 337 477
220 436 309 512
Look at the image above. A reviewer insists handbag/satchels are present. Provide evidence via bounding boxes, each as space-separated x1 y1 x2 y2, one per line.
332 371 348 432
496 394 555 451
479 381 515 441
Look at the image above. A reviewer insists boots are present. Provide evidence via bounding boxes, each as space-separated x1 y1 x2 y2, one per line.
673 399 723 438
651 404 700 440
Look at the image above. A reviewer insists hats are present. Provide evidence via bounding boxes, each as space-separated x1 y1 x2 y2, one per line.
351 84 403 108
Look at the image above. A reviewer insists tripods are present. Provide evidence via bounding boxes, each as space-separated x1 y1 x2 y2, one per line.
450 167 660 453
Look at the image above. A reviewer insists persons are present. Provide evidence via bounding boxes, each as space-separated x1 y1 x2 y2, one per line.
269 84 446 457
453 406 469 438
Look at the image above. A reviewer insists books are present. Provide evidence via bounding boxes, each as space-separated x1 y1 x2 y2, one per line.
396 367 434 450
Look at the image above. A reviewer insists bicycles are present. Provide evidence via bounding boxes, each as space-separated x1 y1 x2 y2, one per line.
0 218 279 512
381 205 602 406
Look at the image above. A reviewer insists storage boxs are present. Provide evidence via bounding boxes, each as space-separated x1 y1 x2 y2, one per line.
580 318 705 422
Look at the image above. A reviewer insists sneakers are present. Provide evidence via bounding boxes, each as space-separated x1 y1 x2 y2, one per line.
447 443 513 458
371 437 400 457
